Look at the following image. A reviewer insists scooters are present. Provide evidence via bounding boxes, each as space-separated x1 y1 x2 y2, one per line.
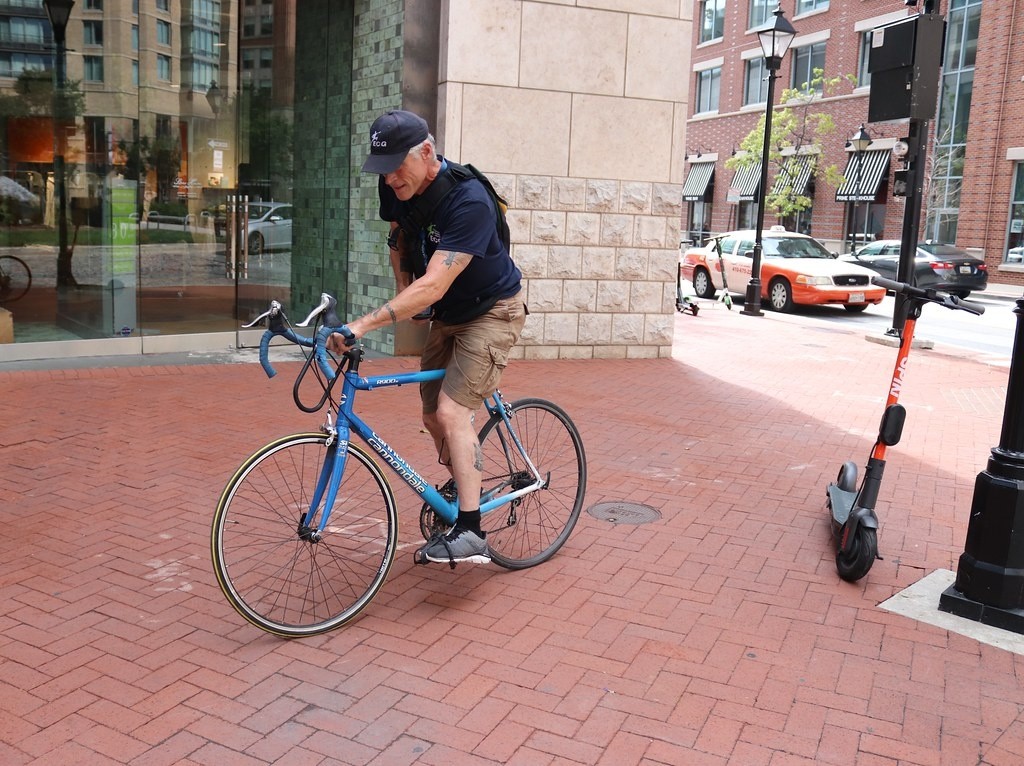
695 232 733 310
675 239 699 315
825 273 987 590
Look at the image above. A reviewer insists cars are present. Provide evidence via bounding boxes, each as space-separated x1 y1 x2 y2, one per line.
1007 247 1024 264
47 170 103 200
681 225 886 315
848 232 877 243
837 239 989 299
241 202 293 255
1 170 45 200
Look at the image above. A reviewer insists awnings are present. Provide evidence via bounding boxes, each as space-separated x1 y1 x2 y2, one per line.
770 155 816 202
835 150 891 202
682 162 715 201
730 160 762 201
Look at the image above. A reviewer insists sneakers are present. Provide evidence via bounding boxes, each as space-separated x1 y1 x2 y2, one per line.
419 520 492 565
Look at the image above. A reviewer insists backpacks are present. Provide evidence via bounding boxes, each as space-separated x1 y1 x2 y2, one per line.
386 163 511 280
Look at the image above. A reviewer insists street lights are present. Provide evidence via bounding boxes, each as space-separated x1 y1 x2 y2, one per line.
740 2 801 315
206 80 225 140
848 123 873 253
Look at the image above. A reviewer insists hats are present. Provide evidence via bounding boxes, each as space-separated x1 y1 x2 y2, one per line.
360 110 429 174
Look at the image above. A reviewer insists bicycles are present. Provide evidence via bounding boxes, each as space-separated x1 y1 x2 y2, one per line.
208 293 588 640
0 253 33 305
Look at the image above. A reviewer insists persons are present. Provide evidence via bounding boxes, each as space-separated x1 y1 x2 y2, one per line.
148 196 196 300
324 110 531 564
105 164 125 189
